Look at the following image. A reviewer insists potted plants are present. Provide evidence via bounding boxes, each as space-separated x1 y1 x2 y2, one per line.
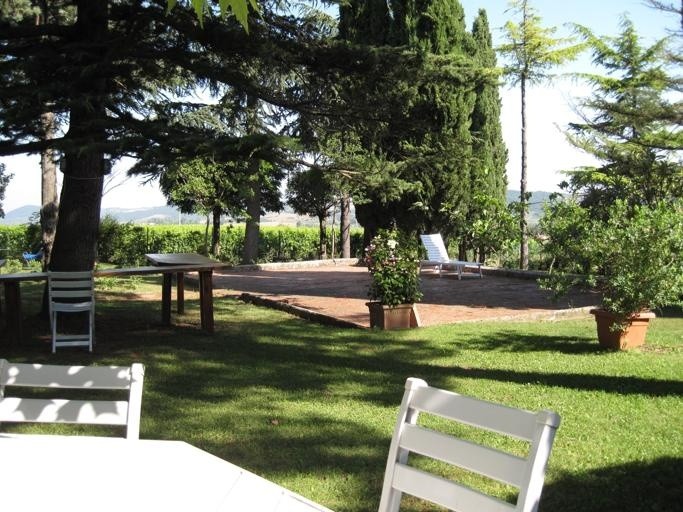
581 196 683 352
365 227 424 330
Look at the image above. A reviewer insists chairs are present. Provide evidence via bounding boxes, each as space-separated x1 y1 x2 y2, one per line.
419 233 484 281
0 357 146 441
46 270 96 355
377 377 561 511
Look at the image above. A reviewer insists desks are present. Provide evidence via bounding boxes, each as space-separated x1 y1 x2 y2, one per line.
145 253 221 331
1 435 332 512
1 261 234 354
